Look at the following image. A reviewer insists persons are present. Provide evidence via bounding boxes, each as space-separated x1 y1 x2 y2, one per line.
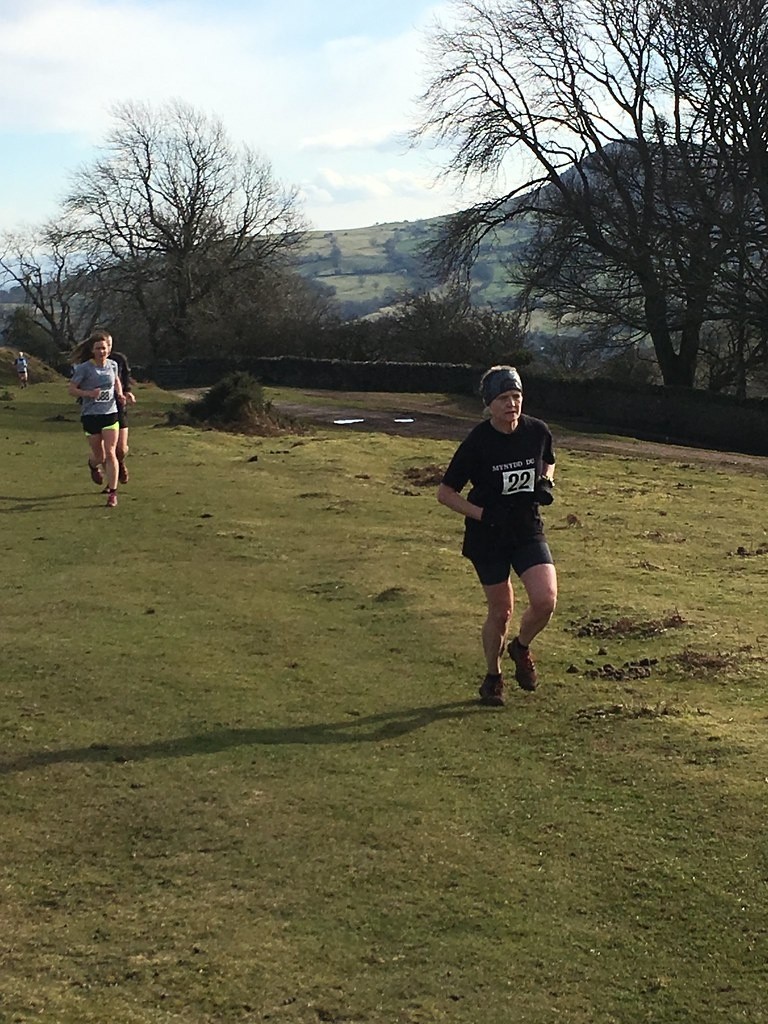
69 334 126 507
14 352 29 389
437 366 557 705
76 335 136 484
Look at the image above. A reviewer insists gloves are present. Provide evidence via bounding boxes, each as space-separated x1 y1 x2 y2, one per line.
481 504 512 525
535 474 555 506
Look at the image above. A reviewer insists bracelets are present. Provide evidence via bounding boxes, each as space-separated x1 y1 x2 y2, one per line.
538 475 555 486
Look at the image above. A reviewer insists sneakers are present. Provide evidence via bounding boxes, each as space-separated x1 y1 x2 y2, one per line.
88 460 103 485
119 459 128 484
508 637 538 691
108 493 117 507
479 672 504 706
